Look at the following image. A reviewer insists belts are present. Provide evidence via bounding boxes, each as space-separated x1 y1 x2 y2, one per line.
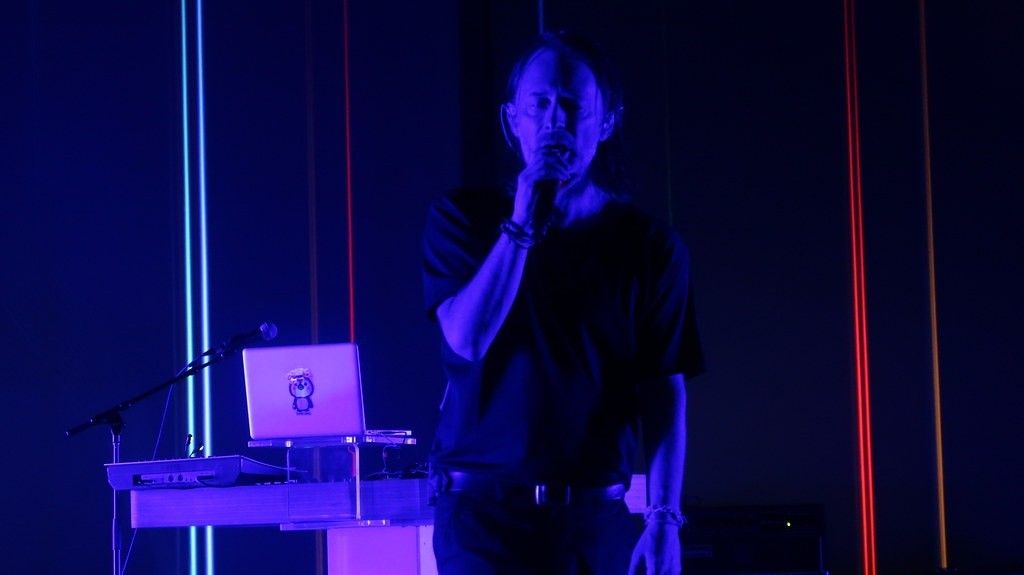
434 468 627 507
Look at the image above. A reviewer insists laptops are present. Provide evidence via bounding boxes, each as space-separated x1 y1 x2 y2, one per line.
242 343 412 436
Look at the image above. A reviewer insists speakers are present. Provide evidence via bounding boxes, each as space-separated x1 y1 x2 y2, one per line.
678 499 831 575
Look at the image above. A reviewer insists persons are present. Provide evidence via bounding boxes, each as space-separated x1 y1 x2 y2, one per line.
424 32 706 575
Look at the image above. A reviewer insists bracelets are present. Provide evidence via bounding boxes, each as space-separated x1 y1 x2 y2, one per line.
645 505 684 527
499 218 538 250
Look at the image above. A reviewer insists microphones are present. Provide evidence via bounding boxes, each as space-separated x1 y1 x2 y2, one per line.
531 143 570 238
204 322 278 357
